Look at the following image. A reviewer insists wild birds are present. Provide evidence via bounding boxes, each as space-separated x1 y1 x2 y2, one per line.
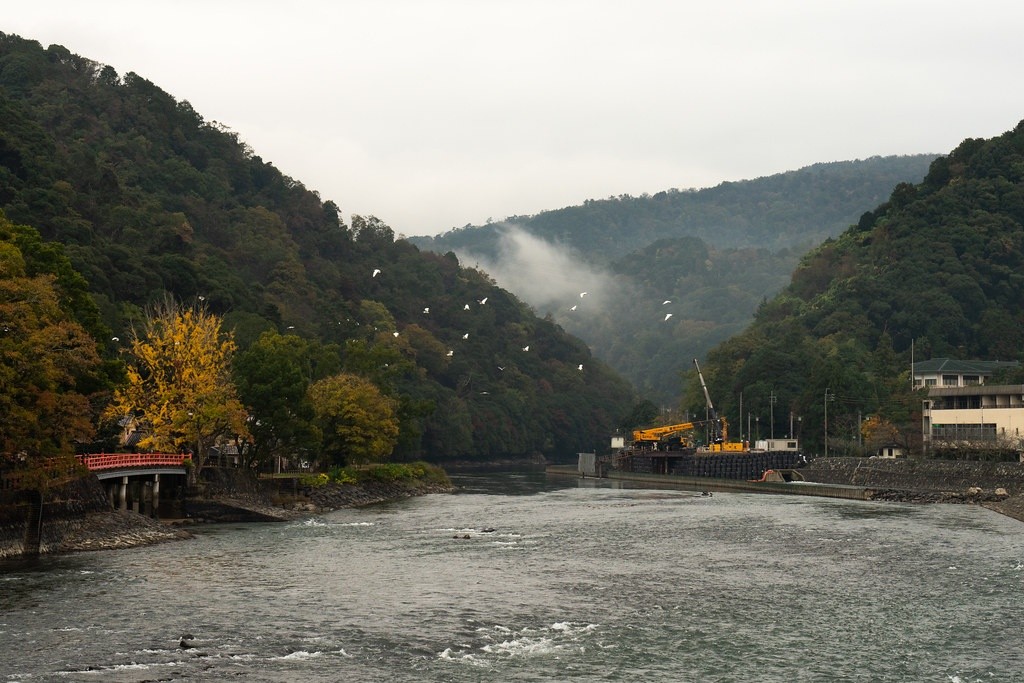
108 266 674 396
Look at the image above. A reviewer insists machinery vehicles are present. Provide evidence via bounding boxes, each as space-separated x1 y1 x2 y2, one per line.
631 357 744 453
